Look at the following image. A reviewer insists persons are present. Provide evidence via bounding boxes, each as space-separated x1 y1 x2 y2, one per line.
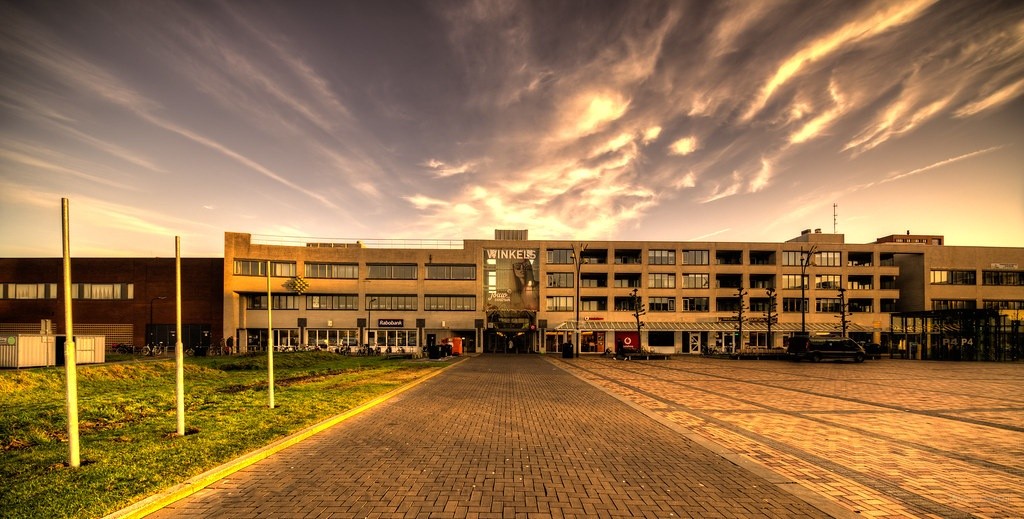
616 338 623 357
510 257 539 311
508 339 513 353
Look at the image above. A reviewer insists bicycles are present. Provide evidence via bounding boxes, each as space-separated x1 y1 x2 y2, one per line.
280 343 405 357
109 343 164 357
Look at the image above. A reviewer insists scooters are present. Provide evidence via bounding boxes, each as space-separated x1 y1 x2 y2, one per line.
186 342 222 357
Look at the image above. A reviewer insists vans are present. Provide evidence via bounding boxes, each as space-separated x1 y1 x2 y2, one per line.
805 339 867 364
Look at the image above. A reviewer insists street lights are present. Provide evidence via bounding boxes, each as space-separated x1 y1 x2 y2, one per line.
367 300 375 356
149 297 167 358
799 245 818 336
570 243 589 358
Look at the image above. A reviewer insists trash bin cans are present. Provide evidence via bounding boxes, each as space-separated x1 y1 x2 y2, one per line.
562 342 573 358
910 343 922 360
428 344 452 359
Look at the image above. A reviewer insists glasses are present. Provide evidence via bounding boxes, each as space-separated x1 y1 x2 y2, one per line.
513 261 530 270
